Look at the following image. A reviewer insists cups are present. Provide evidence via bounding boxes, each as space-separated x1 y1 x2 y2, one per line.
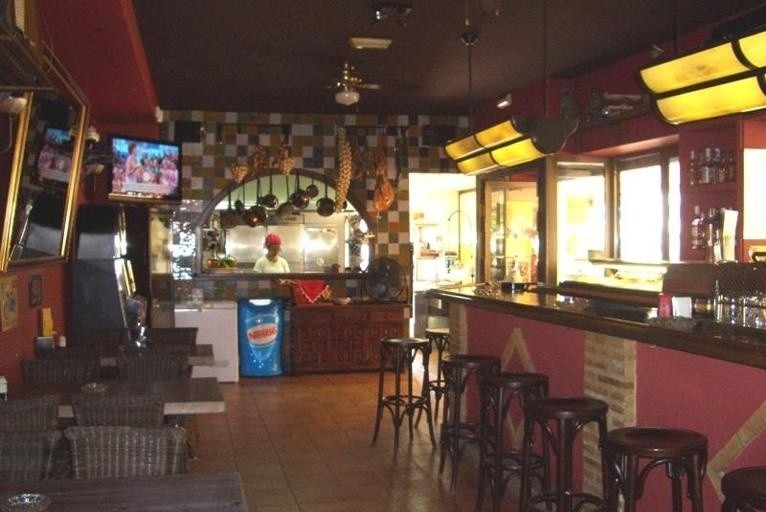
502 283 512 288
713 293 766 332
514 283 529 292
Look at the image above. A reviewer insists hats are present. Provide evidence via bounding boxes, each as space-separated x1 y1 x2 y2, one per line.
265 235 280 248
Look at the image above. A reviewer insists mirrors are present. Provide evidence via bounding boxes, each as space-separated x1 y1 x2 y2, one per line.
0 87 88 273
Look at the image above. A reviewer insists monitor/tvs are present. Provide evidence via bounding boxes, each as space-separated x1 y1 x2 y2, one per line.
107 132 182 205
30 125 76 194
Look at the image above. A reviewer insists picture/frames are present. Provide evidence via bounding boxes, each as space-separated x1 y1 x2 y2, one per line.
28 274 43 308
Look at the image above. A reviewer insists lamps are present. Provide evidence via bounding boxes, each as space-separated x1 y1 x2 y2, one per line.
369 0 412 29
334 85 361 107
636 0 766 126
444 0 579 176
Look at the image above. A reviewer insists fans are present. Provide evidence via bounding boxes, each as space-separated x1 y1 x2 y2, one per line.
360 258 409 303
323 60 382 113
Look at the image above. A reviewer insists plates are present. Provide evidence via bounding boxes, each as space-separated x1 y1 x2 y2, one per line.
79 382 109 394
208 267 239 274
6 491 52 511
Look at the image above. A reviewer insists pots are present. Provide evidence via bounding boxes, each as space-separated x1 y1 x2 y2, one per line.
221 174 347 229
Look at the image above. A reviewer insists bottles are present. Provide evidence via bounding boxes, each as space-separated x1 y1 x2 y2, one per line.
693 298 713 314
692 205 734 248
0 375 8 402
10 197 35 260
58 335 66 347
657 292 672 318
688 147 737 186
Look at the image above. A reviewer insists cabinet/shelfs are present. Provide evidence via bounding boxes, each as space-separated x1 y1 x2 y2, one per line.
678 116 744 264
282 299 413 377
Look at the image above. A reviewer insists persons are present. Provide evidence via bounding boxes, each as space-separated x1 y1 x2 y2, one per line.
254 232 290 273
38 142 72 183
112 143 178 199
345 216 365 272
